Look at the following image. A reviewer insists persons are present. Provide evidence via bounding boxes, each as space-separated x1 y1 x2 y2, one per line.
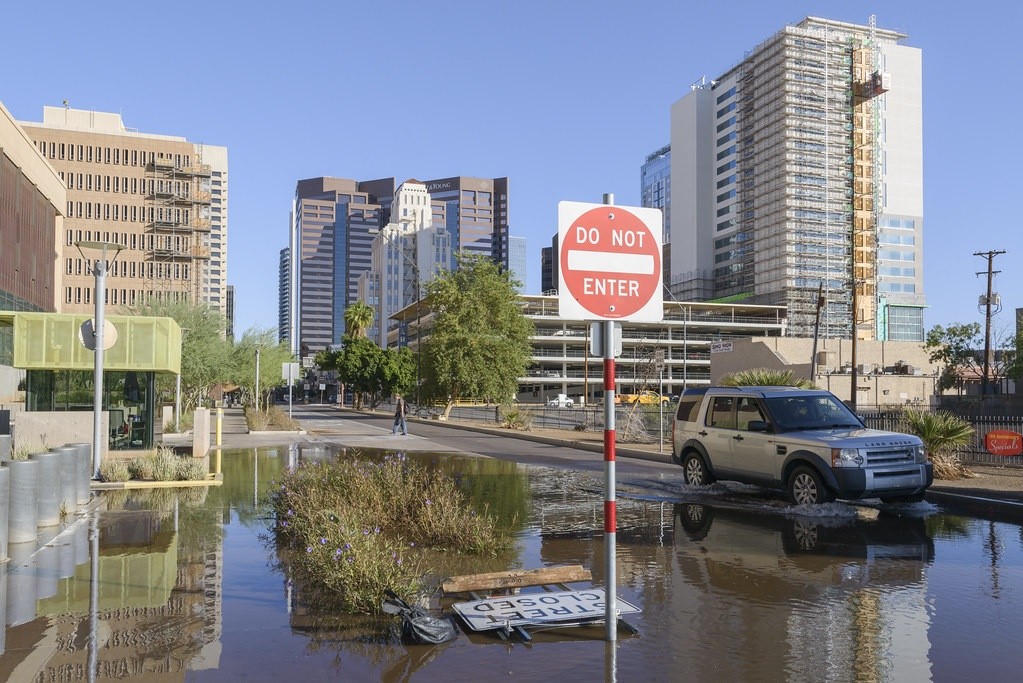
779 401 808 423
390 393 407 436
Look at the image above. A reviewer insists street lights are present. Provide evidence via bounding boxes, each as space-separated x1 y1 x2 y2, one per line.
368 228 422 418
73 240 130 481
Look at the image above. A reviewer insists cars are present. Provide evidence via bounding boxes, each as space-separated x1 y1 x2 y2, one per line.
545 396 574 407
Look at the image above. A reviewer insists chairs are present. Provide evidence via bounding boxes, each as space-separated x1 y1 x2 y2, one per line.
798 403 817 420
742 405 756 412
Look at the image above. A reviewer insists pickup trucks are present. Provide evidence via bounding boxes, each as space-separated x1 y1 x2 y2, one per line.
620 390 671 408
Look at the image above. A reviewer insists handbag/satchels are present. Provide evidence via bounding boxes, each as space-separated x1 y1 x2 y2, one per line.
404 401 411 414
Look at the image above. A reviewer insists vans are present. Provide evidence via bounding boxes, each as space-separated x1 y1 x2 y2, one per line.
599 393 622 406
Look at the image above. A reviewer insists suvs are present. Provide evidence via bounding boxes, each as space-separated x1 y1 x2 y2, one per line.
671 386 934 509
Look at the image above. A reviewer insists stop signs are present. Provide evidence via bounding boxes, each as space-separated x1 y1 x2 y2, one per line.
557 200 664 324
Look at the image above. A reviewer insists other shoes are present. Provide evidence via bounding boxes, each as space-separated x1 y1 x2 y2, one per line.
401 432 406 435
389 432 396 435
